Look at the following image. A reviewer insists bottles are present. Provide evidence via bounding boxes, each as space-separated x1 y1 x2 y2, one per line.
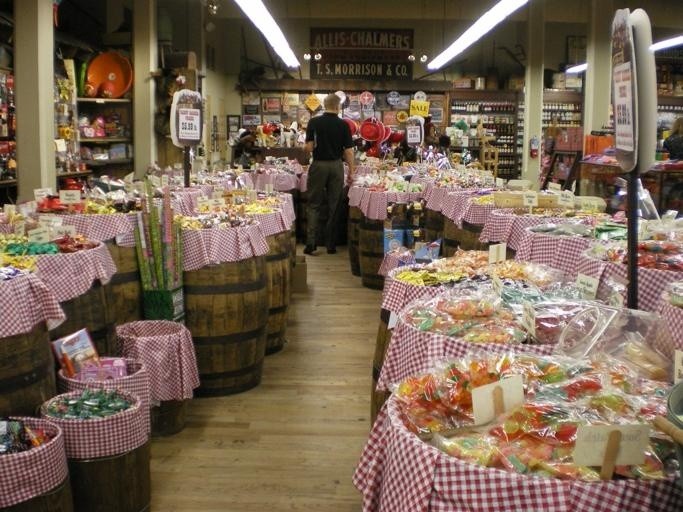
658 105 682 129
518 101 581 180
450 99 514 180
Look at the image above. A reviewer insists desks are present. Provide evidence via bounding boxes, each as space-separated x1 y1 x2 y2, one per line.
641 168 683 217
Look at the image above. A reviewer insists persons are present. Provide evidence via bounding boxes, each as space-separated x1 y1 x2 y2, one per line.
303 94 355 254
661 117 683 214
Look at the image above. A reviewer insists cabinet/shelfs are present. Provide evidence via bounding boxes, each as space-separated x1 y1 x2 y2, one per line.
448 88 583 180
652 33 683 107
0 12 134 184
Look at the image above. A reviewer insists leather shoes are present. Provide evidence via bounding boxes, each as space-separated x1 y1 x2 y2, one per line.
304 245 316 254
327 247 337 253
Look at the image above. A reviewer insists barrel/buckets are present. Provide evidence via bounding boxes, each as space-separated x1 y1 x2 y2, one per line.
3 172 298 510
349 175 682 510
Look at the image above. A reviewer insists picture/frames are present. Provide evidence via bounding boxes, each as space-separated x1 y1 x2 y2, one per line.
226 115 241 140
565 34 586 64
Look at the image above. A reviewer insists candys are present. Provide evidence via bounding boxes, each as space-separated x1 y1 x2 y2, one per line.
0 183 142 283
395 236 683 481
172 195 280 229
0 389 133 458
